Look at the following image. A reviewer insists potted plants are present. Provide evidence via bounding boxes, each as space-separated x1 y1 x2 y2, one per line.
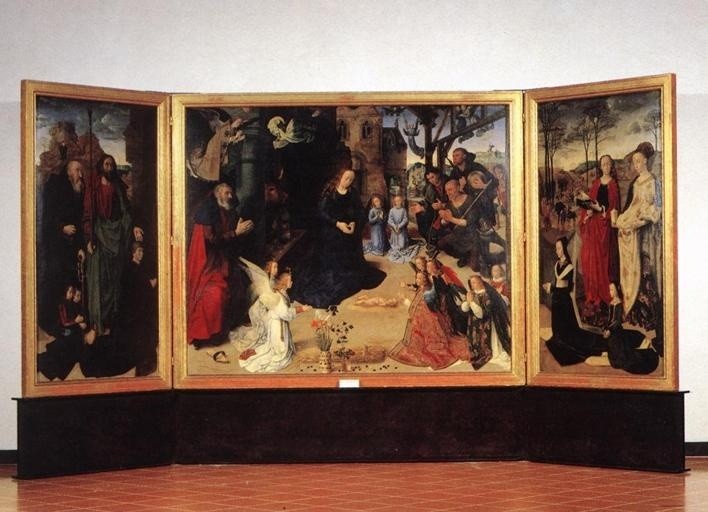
172 89 525 389
21 79 172 397
525 72 678 392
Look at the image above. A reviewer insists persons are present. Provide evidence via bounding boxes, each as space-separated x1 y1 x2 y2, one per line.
186 106 511 372
32 121 158 381
542 142 662 373
